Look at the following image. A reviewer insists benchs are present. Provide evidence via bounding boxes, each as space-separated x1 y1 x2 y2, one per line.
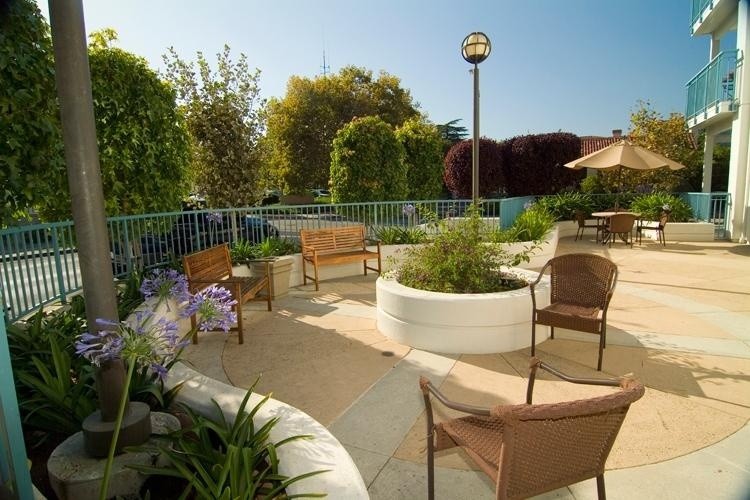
182 242 275 345
300 225 381 290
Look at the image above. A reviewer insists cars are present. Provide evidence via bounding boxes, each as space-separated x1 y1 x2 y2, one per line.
110 213 278 284
313 189 331 197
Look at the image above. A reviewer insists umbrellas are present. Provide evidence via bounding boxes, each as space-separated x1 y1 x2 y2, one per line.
562 139 687 212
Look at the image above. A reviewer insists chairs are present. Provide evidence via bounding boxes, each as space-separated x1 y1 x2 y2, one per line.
530 253 617 370
574 207 670 249
419 357 644 500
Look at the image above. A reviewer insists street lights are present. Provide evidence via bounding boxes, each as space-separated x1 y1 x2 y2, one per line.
462 32 491 214
